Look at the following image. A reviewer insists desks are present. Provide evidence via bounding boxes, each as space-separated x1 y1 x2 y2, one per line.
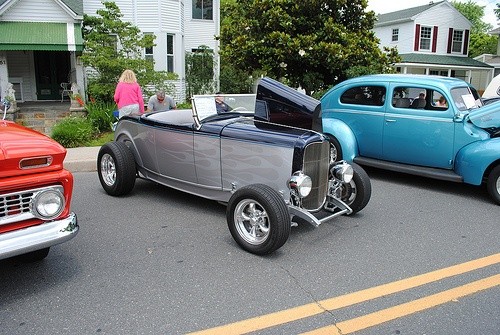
9 77 25 103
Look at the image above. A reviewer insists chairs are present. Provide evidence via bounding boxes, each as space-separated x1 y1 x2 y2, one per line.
411 99 426 109
59 83 73 104
395 98 410 108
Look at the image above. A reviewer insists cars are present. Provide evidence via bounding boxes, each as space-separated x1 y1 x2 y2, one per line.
319 73 500 205
96 76 371 257
1 118 80 264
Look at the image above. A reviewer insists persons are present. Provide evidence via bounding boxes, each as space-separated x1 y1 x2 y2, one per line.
214 92 227 113
419 93 424 99
114 70 144 120
146 90 176 112
435 96 447 107
